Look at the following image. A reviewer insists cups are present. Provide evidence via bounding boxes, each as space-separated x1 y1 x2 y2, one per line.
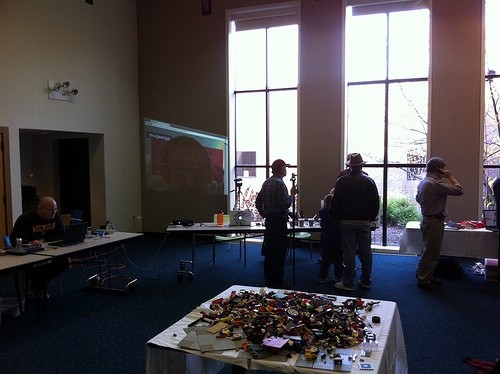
98 232 105 236
288 219 293 227
308 219 315 227
298 219 305 227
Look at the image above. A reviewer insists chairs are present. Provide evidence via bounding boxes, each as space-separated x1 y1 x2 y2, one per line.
3 236 73 304
287 222 314 262
213 215 247 268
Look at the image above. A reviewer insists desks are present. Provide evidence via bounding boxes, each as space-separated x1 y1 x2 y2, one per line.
166 221 379 281
146 285 397 374
0 232 143 330
399 220 500 258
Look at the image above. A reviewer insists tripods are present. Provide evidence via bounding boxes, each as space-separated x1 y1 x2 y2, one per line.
230 187 250 211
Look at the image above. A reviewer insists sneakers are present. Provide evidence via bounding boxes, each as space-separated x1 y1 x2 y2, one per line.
312 271 325 283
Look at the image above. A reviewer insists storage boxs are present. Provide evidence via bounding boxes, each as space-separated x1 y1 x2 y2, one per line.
0 298 25 317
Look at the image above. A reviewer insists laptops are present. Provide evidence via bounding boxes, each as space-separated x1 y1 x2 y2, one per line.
483 210 499 231
6 246 44 255
229 211 251 225
48 222 88 246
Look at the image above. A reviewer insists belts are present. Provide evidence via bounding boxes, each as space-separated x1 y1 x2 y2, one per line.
423 214 445 221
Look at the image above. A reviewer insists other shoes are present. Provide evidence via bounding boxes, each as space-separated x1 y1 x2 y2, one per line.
15 298 25 317
270 282 293 289
418 279 444 290
38 289 49 299
333 275 354 291
358 279 372 288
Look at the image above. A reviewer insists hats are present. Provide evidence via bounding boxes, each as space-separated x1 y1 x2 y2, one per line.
426 157 445 177
346 153 364 167
272 160 290 171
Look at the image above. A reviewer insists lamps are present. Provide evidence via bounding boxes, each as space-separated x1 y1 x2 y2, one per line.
48 80 80 102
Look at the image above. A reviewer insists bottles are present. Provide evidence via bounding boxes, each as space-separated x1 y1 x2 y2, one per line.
217 210 224 225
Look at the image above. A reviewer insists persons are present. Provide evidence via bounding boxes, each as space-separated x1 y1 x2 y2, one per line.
256 159 297 288
319 153 380 290
9 196 65 300
416 158 464 288
